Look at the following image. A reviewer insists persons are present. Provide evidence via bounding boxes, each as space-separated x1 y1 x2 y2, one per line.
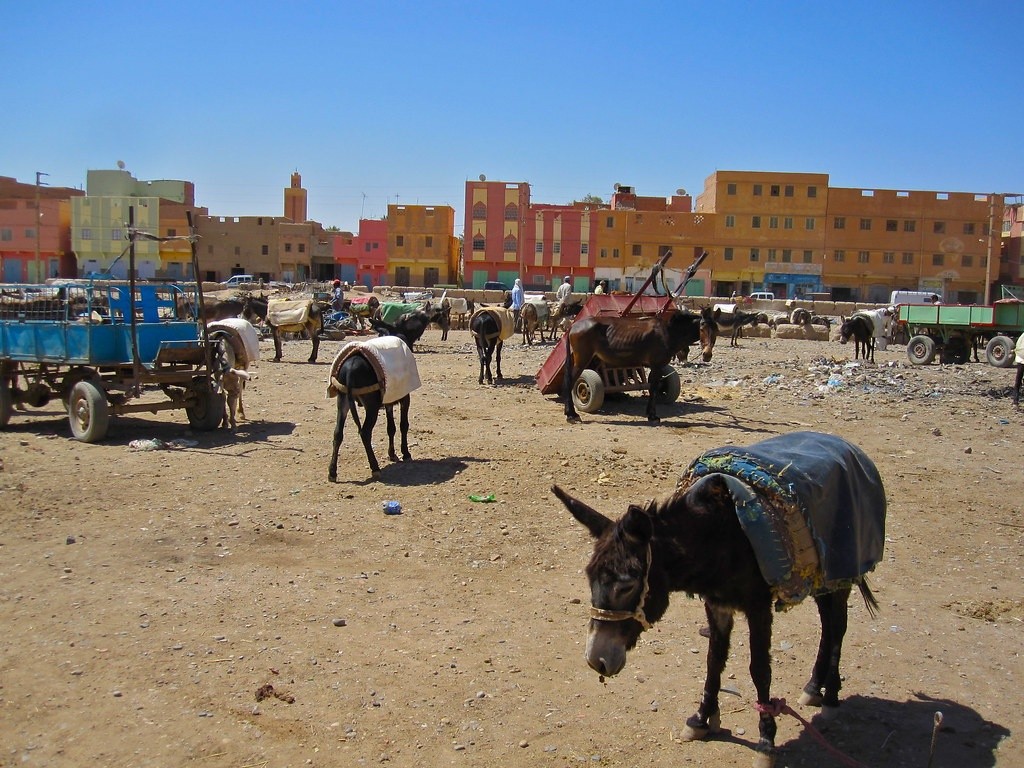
479 290 513 309
556 276 572 332
352 280 357 286
931 295 943 305
512 279 524 334
344 280 349 292
594 280 606 295
1010 332 1024 408
330 279 344 313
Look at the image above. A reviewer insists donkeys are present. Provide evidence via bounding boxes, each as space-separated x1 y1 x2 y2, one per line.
0 279 902 490
551 431 885 768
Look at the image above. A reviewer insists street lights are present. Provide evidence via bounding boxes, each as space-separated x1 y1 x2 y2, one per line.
35 171 50 284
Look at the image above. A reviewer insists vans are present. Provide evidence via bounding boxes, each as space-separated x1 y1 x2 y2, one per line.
891 290 945 306
484 281 510 290
750 292 775 300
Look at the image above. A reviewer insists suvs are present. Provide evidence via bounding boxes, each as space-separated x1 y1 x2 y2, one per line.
220 274 255 288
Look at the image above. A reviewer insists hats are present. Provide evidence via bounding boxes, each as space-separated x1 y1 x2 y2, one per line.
599 280 605 285
888 307 894 314
333 280 341 285
564 276 570 281
